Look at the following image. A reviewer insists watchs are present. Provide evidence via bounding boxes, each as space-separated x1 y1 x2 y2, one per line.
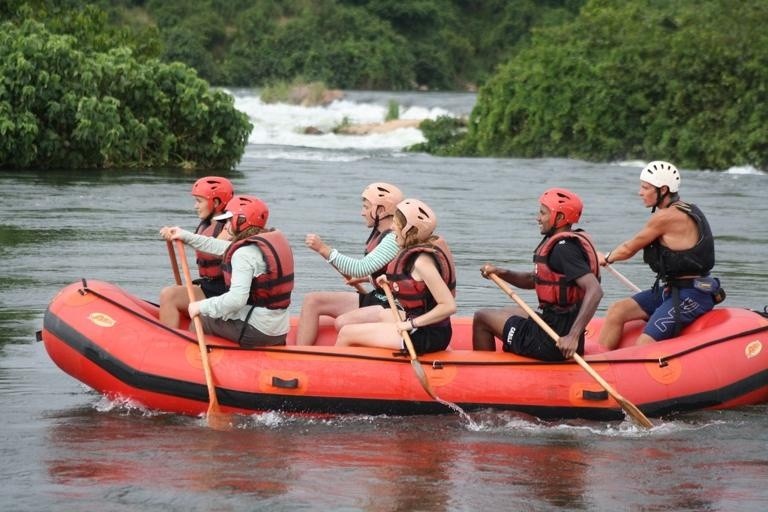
605 250 614 264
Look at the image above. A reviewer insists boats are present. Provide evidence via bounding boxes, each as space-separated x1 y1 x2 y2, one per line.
34 276 768 422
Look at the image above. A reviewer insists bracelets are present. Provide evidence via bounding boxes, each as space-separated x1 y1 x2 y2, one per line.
410 318 417 329
326 248 338 263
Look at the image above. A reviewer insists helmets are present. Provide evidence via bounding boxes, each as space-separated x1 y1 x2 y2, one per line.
640 160 680 194
362 182 403 229
397 198 437 242
539 187 584 228
212 194 270 233
192 176 234 213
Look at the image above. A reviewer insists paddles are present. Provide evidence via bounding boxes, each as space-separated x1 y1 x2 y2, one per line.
485 265 653 429
177 242 222 429
382 282 436 399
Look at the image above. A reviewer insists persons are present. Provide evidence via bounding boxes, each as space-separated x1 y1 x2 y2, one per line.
158 176 234 330
295 183 405 346
165 195 295 347
597 160 726 352
335 198 457 355
472 188 604 361
